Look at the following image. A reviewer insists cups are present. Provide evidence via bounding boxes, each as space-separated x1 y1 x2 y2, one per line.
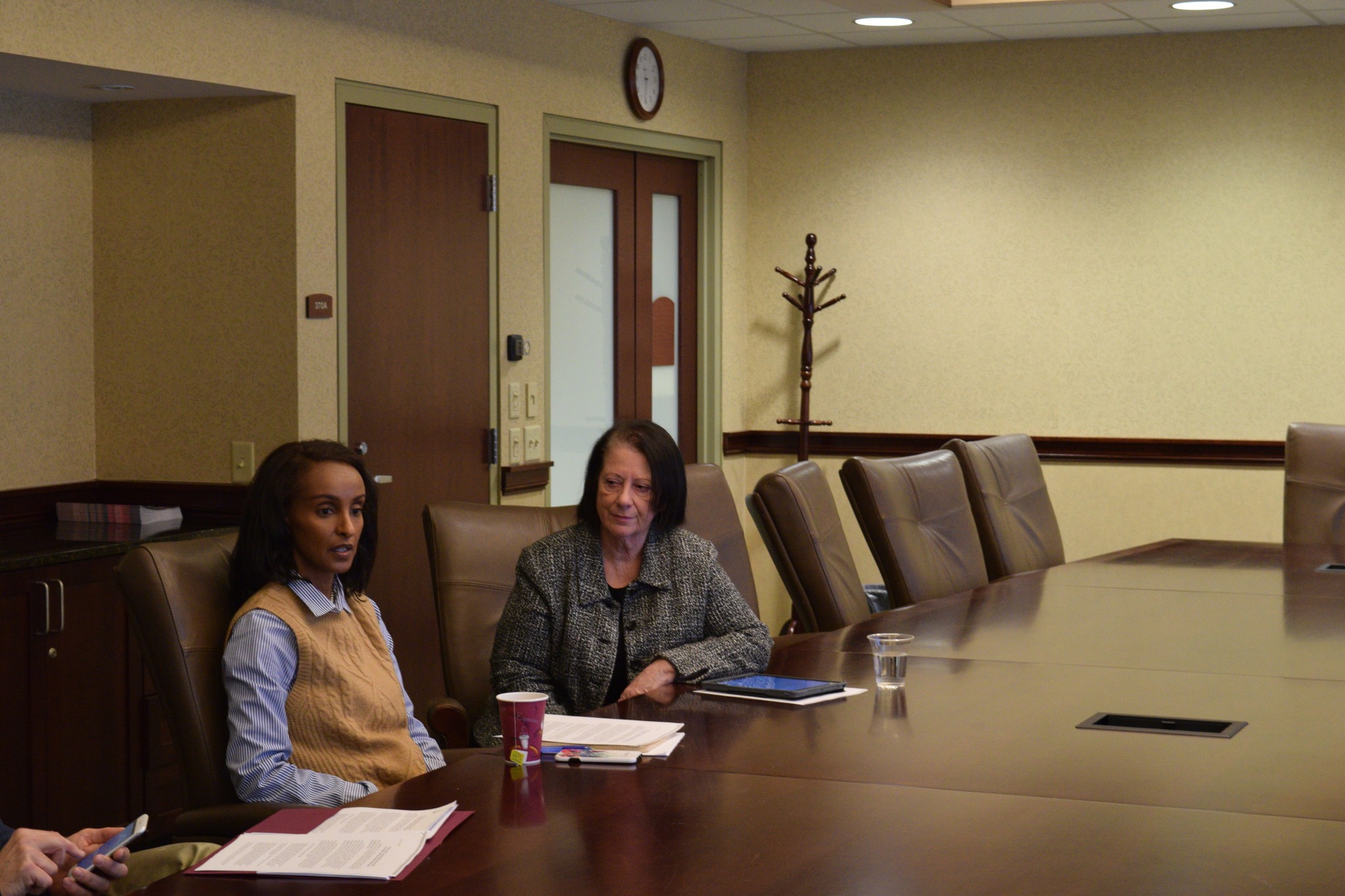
867 633 916 689
496 691 549 766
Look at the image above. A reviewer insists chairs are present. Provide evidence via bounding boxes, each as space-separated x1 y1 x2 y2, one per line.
422 502 588 747
744 428 1067 636
1281 422 1344 543
675 460 766 630
124 532 308 838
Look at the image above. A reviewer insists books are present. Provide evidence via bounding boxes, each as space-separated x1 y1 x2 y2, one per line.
56 499 184 525
490 712 686 755
56 519 182 543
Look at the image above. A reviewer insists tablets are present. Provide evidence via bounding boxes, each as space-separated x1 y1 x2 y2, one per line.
701 671 844 700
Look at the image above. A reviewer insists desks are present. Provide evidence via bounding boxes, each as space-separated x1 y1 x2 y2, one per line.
91 524 1345 891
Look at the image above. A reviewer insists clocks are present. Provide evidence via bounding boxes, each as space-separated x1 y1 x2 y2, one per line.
626 36 666 118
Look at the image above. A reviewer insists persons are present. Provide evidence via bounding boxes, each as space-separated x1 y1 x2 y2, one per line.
533 714 766 896
0 810 224 896
473 418 774 752
218 434 448 806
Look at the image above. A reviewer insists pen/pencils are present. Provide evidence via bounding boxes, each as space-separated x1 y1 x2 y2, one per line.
542 745 591 752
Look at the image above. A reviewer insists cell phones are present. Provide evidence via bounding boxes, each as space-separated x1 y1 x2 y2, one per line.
555 749 644 764
67 814 149 883
